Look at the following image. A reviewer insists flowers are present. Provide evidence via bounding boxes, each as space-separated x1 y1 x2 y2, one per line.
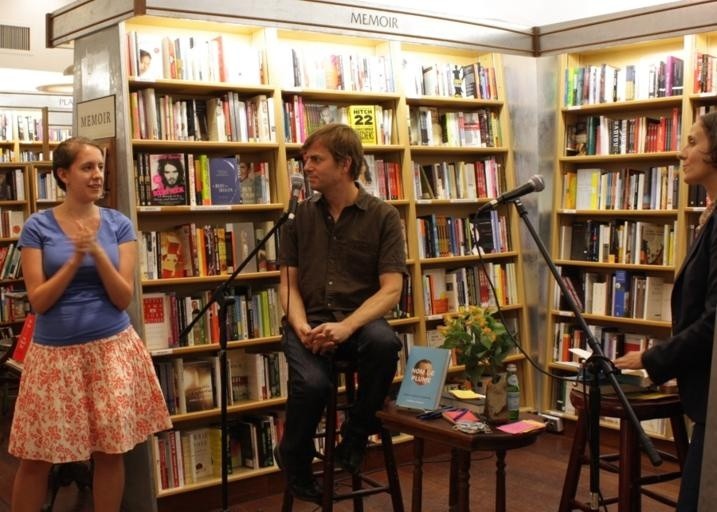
437 297 515 378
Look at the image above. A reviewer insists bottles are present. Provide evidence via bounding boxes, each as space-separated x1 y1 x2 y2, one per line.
504 365 521 421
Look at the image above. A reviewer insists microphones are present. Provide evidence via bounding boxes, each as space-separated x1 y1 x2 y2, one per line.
479 174 545 213
287 173 304 227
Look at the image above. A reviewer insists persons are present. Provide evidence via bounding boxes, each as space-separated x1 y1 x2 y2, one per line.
1 135 178 511
612 108 716 511
275 124 409 498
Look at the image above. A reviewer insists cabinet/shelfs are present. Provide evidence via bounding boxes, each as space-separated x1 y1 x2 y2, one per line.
119 17 539 512
541 27 716 433
0 95 75 338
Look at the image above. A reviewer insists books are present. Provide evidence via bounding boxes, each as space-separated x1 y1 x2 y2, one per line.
126 29 288 490
0 109 74 381
402 56 521 412
284 47 418 450
553 54 716 437
396 346 451 411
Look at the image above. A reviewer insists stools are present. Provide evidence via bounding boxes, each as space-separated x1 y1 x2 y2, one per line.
281 357 401 511
557 381 690 511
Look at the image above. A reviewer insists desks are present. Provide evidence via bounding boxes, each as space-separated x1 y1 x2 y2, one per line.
377 395 544 510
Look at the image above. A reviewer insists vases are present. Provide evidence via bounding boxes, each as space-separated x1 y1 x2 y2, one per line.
486 371 508 421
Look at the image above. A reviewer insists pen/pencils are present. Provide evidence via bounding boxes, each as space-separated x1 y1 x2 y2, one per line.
415 405 454 420
453 410 468 420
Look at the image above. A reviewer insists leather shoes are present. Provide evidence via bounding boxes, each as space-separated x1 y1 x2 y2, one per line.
273 446 323 502
333 418 368 472
